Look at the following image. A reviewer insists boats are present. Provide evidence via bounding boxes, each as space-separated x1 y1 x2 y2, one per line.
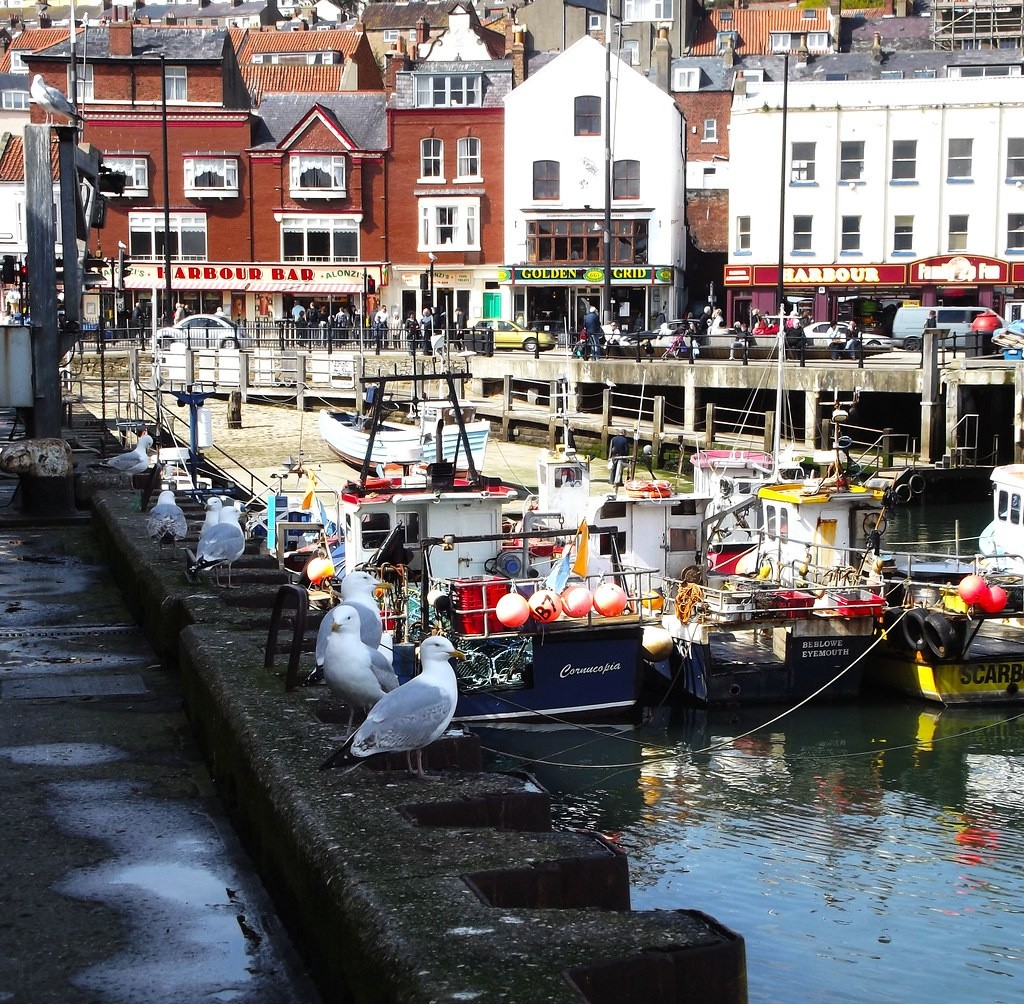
263 301 1024 728
316 292 491 481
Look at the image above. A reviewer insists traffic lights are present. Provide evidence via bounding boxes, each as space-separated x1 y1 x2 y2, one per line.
0 255 16 285
118 250 131 290
20 265 27 279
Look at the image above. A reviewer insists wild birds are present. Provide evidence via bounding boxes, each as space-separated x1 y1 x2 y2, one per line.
191 506 246 588
198 497 222 538
90 435 154 490
31 74 87 124
315 571 392 737
428 252 437 262
323 605 400 708
118 240 126 249
319 635 466 781
147 491 187 559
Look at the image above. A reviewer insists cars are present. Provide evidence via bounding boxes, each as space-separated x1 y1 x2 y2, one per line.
802 321 894 357
469 318 557 352
148 312 253 351
657 319 734 337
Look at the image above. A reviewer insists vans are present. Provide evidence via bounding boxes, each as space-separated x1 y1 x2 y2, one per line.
891 305 1006 353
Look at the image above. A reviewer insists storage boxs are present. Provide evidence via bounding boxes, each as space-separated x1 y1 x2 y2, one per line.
771 591 816 618
828 589 886 617
446 575 508 634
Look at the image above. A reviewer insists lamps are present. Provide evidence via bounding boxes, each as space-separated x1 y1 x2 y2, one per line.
392 494 403 504
507 490 517 500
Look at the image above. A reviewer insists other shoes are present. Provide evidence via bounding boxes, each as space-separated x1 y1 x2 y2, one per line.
618 483 624 486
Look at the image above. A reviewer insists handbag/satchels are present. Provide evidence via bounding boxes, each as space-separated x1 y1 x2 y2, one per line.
608 459 614 470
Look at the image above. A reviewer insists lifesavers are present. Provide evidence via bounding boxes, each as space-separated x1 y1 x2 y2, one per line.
895 474 926 504
625 479 672 498
366 477 394 488
885 607 958 659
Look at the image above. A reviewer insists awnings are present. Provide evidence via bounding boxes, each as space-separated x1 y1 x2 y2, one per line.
95 281 364 293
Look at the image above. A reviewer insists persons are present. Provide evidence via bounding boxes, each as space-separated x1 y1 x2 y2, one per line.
784 319 805 359
568 426 577 452
572 307 620 360
291 299 359 347
609 429 630 486
787 312 811 328
390 312 402 349
924 310 936 328
215 307 224 327
655 310 665 329
846 321 856 359
643 340 654 357
454 309 466 352
4 310 12 325
752 309 779 335
736 324 753 359
672 313 698 360
173 303 190 325
371 305 388 347
405 308 441 355
117 302 144 342
698 306 740 346
827 321 841 361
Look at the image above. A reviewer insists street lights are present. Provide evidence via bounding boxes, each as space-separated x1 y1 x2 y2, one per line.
428 251 438 356
599 19 636 361
142 47 173 327
771 49 792 325
